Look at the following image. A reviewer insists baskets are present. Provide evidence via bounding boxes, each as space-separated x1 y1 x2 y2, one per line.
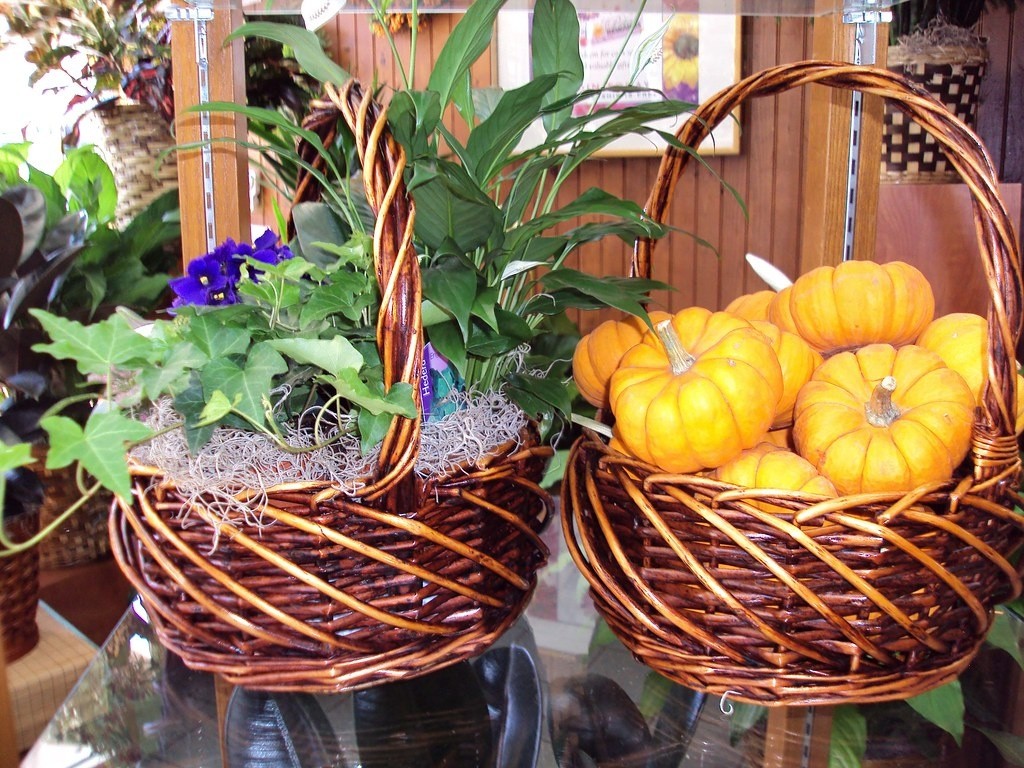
112 80 555 696
558 60 1024 706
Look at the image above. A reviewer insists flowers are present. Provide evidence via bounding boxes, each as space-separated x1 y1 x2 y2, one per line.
160 237 442 436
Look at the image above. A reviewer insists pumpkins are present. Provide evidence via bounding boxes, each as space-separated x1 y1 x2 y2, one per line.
573 260 1024 621
